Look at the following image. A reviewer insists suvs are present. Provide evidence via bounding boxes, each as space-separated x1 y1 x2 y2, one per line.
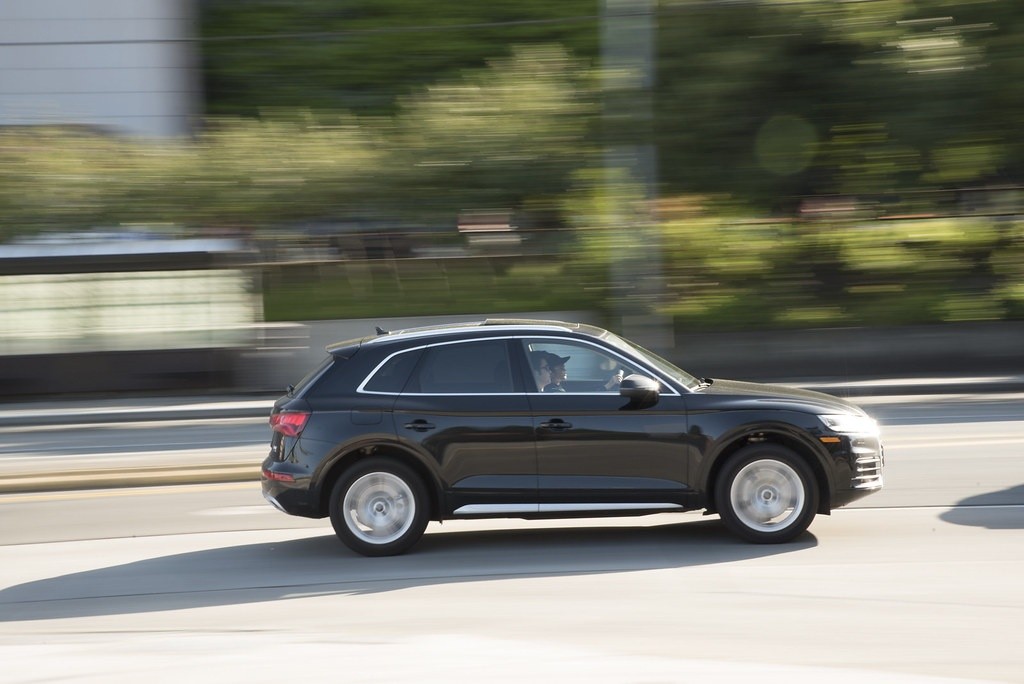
259 318 886 557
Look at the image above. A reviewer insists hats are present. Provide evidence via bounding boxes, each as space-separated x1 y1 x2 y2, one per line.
549 353 570 366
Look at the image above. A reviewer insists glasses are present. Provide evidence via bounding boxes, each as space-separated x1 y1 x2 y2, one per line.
539 365 550 371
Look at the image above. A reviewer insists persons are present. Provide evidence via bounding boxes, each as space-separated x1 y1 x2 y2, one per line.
545 352 622 393
531 350 551 392
